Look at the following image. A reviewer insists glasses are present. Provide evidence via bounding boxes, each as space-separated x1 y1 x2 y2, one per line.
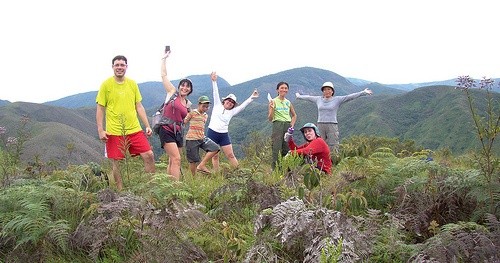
227 99 235 104
112 64 128 68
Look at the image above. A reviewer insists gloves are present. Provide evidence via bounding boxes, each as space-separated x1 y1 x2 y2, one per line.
284 133 293 142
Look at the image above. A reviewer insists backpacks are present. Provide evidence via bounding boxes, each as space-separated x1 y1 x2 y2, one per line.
152 90 191 134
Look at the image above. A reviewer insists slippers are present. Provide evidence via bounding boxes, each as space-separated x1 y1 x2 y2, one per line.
196 169 212 175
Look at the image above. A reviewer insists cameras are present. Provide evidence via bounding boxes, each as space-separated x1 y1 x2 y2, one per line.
254 88 257 94
165 46 170 53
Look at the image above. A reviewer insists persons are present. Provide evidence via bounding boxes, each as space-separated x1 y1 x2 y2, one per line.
184 95 220 176
159 50 193 180
206 72 259 170
96 55 155 191
284 123 332 175
295 82 372 153
268 82 298 175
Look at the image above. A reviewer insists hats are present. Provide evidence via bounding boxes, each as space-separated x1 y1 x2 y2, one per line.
198 95 212 104
299 123 321 142
222 94 239 107
321 82 335 96
